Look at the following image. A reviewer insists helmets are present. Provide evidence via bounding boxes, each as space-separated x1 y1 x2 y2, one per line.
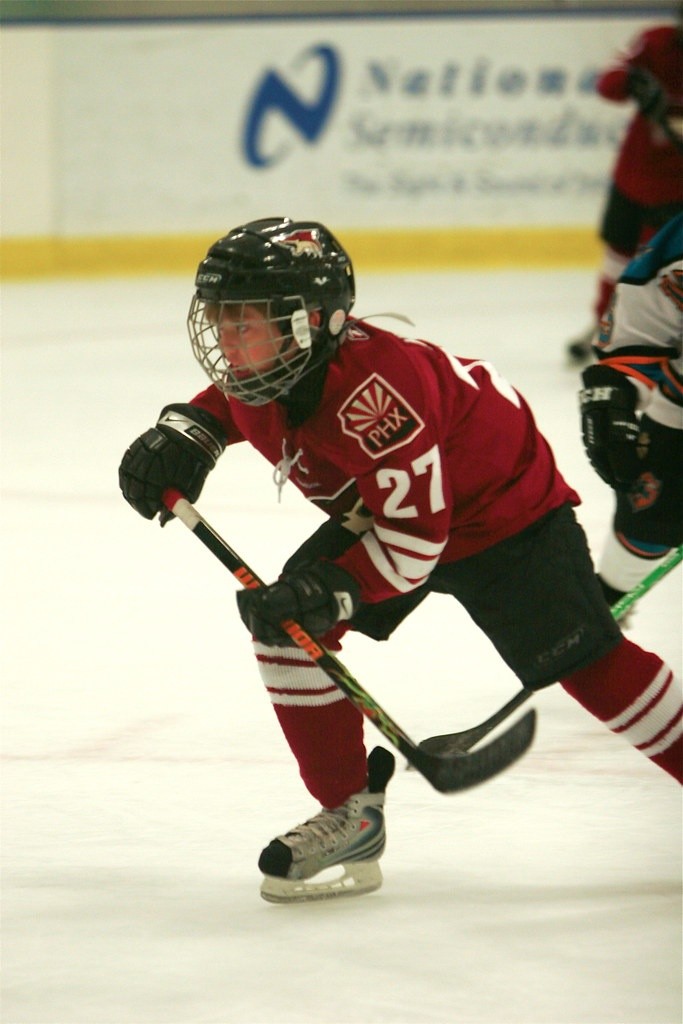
186 218 356 405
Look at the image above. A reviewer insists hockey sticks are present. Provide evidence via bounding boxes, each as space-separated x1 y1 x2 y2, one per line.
405 541 683 773
160 484 539 796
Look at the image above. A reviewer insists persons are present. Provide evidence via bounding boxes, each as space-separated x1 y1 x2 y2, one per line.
577 212 683 626
593 24 683 320
117 217 683 900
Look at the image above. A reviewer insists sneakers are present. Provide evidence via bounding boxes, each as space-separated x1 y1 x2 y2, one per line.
258 746 396 904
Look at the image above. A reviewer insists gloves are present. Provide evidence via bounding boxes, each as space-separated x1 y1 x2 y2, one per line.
118 404 229 528
237 561 360 649
579 364 643 494
626 64 671 124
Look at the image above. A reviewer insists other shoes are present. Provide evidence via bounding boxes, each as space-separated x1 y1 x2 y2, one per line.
565 332 594 369
